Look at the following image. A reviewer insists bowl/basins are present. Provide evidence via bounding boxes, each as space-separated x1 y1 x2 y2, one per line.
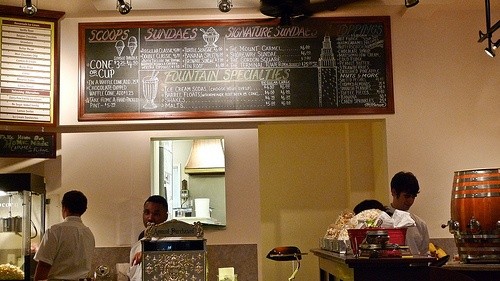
347 227 408 257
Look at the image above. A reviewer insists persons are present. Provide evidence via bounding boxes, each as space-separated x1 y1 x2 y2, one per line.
354 172 432 257
138 194 169 240
33 190 96 281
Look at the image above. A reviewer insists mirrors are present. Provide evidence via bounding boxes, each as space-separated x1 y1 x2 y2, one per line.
150 136 225 229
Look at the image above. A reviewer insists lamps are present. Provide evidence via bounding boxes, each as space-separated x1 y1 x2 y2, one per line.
485 39 500 59
116 0 133 15
217 0 233 13
22 0 37 17
265 246 309 281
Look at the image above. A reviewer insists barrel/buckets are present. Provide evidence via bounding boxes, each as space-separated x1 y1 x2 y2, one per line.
442 168 500 264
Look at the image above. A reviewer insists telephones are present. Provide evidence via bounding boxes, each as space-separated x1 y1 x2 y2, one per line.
75 13 399 121
